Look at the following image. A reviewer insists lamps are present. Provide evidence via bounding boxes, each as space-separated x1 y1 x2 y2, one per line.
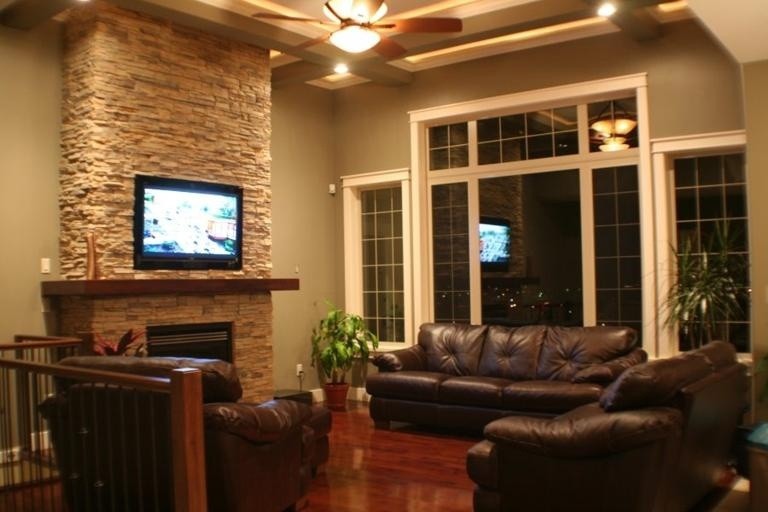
323 0 388 55
590 102 636 151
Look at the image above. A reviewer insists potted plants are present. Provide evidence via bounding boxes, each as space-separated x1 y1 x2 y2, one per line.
310 296 379 409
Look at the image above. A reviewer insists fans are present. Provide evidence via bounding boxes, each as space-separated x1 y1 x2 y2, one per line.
252 0 464 60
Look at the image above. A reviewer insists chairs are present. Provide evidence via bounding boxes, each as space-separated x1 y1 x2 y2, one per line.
36 354 329 512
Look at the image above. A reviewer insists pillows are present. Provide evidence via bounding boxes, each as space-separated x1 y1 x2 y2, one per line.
52 354 242 403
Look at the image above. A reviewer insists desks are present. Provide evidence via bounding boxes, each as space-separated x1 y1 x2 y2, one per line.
274 389 312 404
455 277 540 324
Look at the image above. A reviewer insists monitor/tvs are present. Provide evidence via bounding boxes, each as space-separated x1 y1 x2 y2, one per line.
479 215 513 273
132 173 244 270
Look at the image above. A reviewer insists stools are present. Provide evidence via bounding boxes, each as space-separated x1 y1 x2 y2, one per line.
745 423 768 512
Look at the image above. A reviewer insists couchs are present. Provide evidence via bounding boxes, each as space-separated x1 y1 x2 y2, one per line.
466 343 751 512
368 323 647 437
